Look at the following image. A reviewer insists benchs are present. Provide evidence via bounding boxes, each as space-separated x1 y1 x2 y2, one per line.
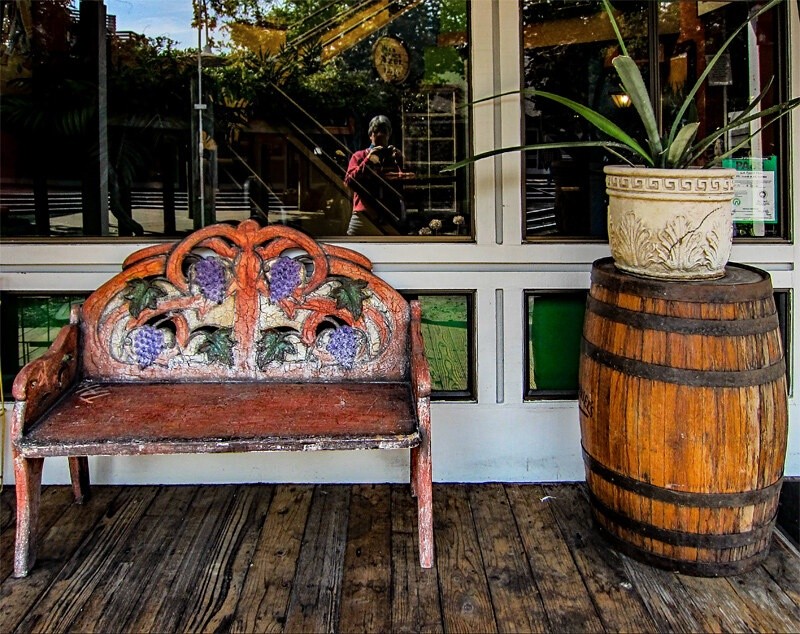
10 219 434 578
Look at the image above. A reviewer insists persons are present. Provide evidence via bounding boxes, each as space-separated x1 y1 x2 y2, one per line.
345 116 415 236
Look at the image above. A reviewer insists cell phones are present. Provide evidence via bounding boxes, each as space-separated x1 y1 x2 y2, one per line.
376 148 392 157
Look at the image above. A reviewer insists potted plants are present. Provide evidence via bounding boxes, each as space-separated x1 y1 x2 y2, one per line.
439 0 800 281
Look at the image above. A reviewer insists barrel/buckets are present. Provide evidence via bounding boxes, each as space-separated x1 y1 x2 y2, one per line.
578 258 789 577
531 295 585 391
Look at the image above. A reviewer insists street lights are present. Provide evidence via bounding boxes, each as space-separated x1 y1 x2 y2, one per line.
609 83 635 153
